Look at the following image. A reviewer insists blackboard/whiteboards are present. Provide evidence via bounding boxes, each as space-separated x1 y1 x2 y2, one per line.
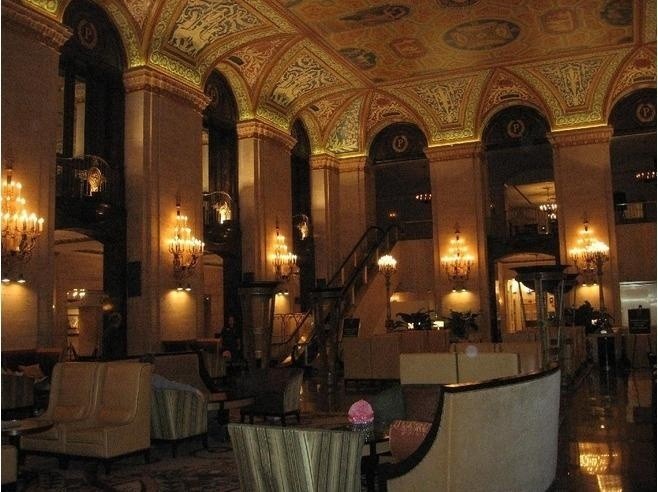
628 308 652 336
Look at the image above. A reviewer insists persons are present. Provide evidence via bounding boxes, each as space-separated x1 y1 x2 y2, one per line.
136 353 206 402
219 314 243 352
204 344 259 427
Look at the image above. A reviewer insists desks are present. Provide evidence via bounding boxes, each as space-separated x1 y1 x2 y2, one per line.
2 361 48 414
3 417 54 487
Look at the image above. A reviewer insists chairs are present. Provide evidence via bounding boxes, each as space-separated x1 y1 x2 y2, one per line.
19 362 154 476
119 351 228 462
228 329 656 492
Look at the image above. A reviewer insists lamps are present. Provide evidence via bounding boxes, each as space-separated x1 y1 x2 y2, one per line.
269 225 302 297
72 159 113 198
376 253 399 303
165 192 209 293
439 223 475 294
1 159 45 287
65 286 88 301
293 212 311 241
567 212 612 288
385 159 657 222
203 189 237 227
